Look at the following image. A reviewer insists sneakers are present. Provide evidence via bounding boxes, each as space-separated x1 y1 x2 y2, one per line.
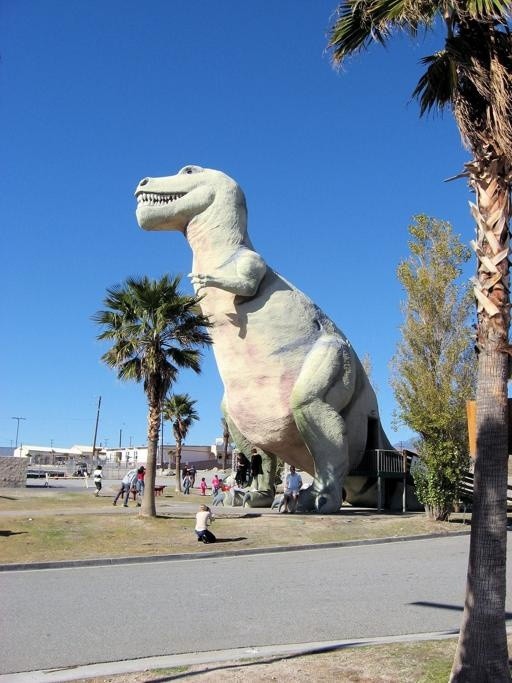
201 535 207 543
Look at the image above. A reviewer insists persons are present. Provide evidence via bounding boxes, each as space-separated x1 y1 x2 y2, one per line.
244 449 264 493
136 465 145 496
92 464 104 497
43 471 51 488
234 451 249 489
182 464 231 507
193 504 216 544
81 471 91 489
111 468 144 507
279 464 303 514
